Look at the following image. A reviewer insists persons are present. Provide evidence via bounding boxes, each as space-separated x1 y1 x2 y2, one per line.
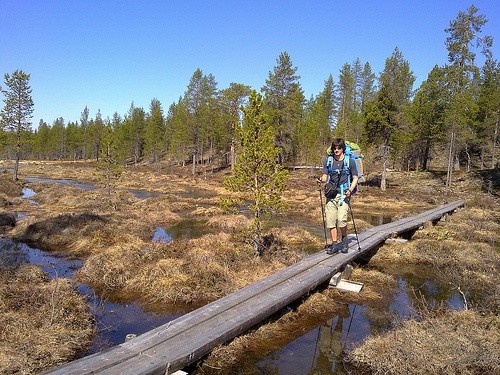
316 138 359 254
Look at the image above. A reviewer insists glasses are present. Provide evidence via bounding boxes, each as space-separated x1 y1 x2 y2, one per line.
332 146 342 150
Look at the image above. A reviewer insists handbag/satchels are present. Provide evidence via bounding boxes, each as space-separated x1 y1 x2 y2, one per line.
324 180 338 199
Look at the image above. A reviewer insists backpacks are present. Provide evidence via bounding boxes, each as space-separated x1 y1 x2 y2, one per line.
326 140 363 206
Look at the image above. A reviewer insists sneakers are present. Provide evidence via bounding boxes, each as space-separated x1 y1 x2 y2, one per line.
327 243 340 254
341 238 349 252
